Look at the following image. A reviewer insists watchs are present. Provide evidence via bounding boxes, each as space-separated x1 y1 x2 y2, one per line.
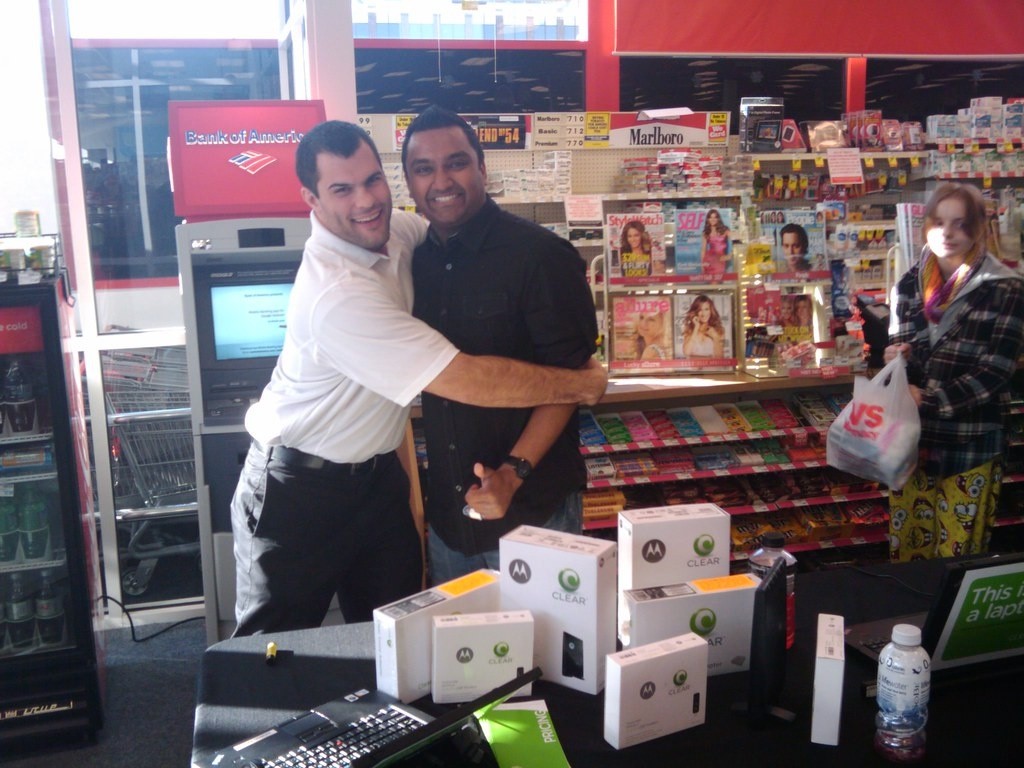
503 453 533 485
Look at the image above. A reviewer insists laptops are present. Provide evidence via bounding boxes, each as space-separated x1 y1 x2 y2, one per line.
844 552 1024 685
193 667 542 768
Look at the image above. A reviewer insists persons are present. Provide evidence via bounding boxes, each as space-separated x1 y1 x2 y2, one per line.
682 295 725 359
630 310 668 360
700 209 734 278
620 221 652 277
780 295 813 349
401 102 600 595
883 183 1024 564
227 120 607 638
780 223 814 274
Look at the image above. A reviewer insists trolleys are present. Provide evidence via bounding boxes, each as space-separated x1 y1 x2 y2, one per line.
80 350 203 595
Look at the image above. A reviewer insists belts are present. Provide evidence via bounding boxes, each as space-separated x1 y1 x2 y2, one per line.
253 437 389 477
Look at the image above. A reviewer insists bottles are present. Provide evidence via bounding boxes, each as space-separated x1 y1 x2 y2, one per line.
0 497 19 561
4 359 34 432
35 571 64 643
749 532 797 648
874 624 930 762
6 573 34 646
0 602 6 648
19 481 50 559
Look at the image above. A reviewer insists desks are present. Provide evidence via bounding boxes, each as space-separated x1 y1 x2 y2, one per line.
190 523 1024 768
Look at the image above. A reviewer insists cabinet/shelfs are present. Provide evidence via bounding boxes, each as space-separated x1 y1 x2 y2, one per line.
400 371 869 586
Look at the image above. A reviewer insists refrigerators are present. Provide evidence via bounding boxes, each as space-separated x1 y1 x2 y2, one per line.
0 270 104 762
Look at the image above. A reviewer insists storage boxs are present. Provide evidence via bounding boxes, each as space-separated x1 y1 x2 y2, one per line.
619 505 731 644
375 567 496 704
499 526 618 693
622 572 755 677
811 613 845 748
430 612 537 704
604 634 710 749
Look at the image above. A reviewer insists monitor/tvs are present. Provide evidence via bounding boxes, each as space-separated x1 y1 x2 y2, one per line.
210 282 294 360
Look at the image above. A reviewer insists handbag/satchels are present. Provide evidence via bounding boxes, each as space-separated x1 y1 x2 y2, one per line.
826 352 919 493
